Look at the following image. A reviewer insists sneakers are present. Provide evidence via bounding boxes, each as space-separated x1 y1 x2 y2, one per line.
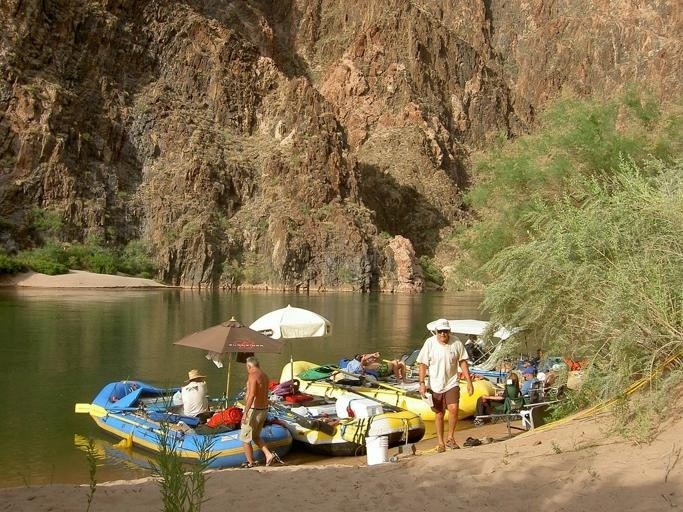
243 455 275 467
439 439 459 452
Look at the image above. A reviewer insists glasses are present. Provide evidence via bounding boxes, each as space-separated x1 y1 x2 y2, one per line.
438 330 448 333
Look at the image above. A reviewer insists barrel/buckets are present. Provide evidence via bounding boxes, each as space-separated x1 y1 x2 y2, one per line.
365 437 388 465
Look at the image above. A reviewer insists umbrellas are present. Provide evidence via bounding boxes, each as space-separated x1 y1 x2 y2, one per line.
246 301 332 382
171 315 282 409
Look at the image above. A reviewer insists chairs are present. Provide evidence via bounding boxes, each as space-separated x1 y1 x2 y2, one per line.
485 373 555 425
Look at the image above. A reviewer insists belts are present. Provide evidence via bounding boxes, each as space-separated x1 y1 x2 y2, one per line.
251 408 268 410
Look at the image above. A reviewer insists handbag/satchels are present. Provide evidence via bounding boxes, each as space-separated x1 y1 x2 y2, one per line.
276 380 299 395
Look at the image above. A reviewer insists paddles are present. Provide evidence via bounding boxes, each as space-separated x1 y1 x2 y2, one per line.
89 404 180 440
74 402 146 413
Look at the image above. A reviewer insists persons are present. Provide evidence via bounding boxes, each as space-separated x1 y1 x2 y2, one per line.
353 350 415 384
415 318 475 453
180 368 209 416
473 372 521 426
524 347 550 378
463 333 489 365
238 356 276 468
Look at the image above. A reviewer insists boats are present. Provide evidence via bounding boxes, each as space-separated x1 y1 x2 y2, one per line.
72 416 197 488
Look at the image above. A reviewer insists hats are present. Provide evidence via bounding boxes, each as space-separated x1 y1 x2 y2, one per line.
436 319 450 330
185 369 205 382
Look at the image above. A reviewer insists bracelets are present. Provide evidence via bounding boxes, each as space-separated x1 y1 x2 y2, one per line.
418 380 425 385
